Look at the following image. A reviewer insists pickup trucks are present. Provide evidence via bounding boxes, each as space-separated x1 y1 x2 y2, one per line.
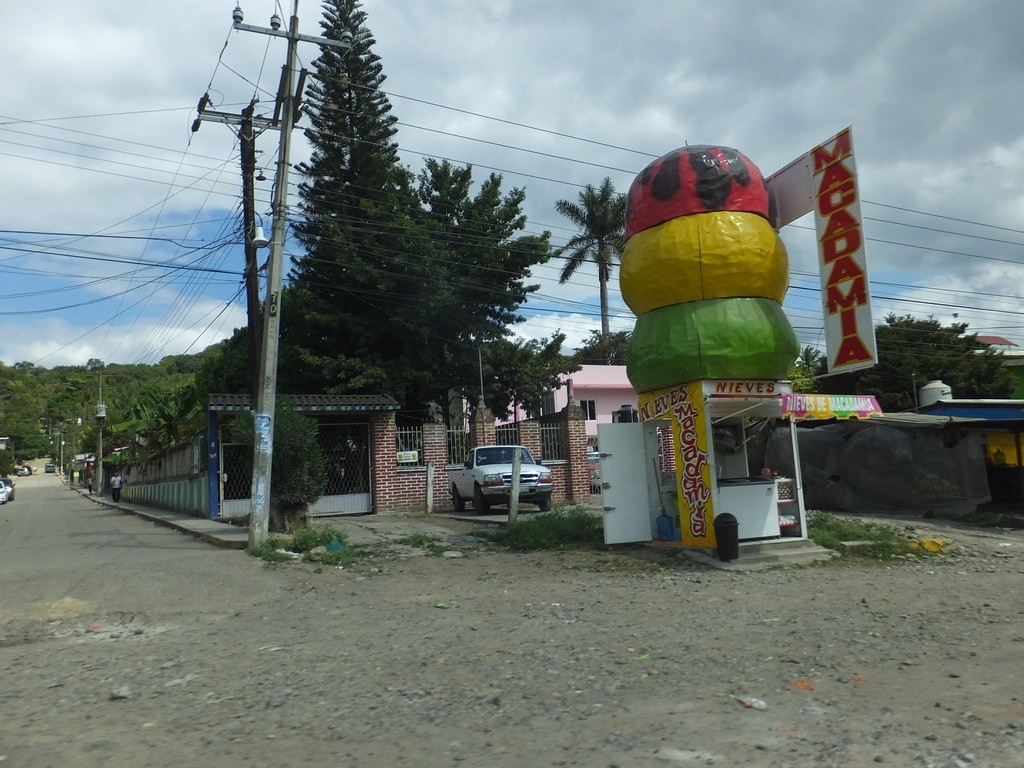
447 444 555 515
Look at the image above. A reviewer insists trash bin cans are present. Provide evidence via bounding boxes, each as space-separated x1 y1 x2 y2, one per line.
713 513 740 562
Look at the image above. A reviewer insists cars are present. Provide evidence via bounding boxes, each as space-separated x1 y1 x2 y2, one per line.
45 464 56 473
17 468 29 478
0 478 16 502
22 466 32 475
0 481 9 504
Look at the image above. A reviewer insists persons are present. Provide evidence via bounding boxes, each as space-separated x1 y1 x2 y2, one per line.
110 471 123 502
86 474 94 495
